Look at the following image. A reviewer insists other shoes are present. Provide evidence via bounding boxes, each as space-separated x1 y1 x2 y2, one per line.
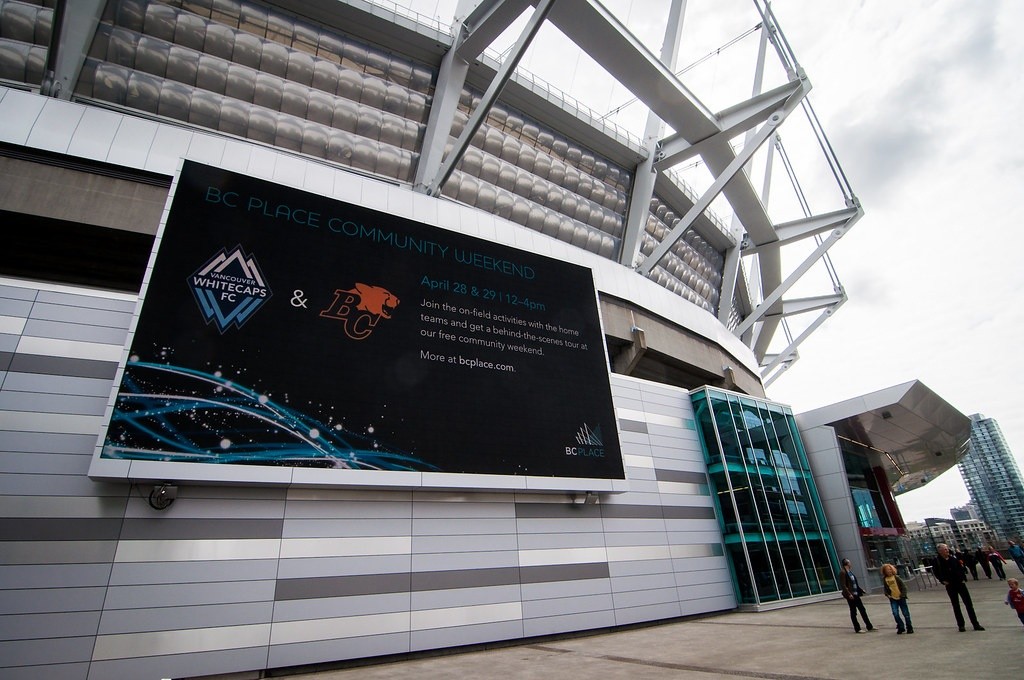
897 629 905 634
973 626 985 631
958 627 965 632
869 627 878 631
906 629 913 634
858 629 866 634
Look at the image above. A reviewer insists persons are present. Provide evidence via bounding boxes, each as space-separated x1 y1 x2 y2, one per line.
954 546 1007 581
840 559 879 633
893 558 907 577
921 555 936 573
880 564 914 634
1008 540 1024 574
932 544 985 632
1004 579 1024 625
